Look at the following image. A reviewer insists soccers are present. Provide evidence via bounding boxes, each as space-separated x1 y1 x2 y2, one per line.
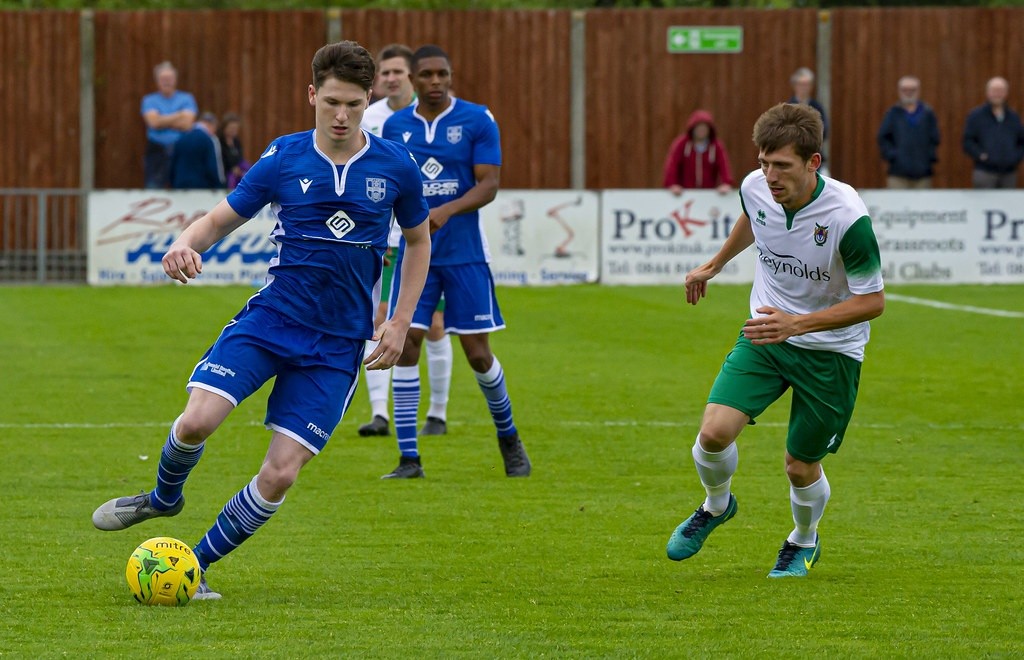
125 536 201 607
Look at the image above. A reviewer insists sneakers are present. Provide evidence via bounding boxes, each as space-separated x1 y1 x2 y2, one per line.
765 533 821 576
91 489 186 532
499 433 531 477
666 491 738 560
192 577 224 599
380 463 425 480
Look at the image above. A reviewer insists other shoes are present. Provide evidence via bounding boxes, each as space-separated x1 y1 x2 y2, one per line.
416 416 447 435
358 415 390 435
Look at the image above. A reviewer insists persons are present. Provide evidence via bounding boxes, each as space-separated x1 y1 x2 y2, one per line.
877 76 941 189
360 44 531 480
92 40 432 602
667 100 886 579
142 60 249 189
962 75 1024 188
785 67 829 171
663 109 733 193
